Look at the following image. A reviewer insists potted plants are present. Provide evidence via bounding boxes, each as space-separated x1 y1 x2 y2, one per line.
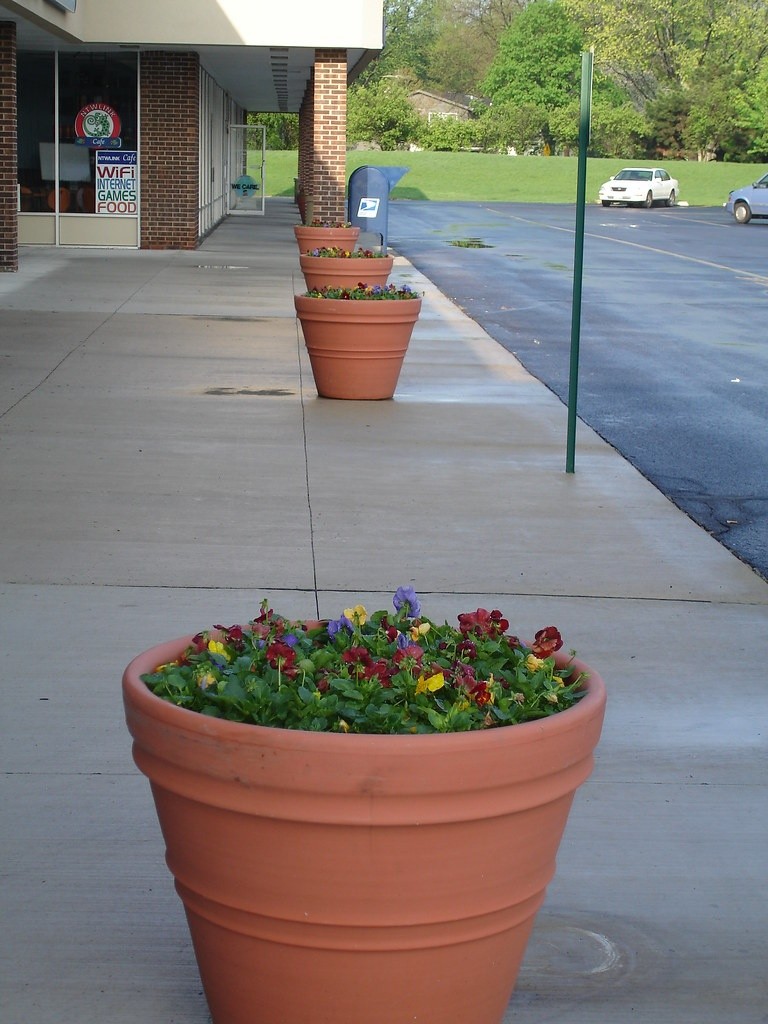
296 184 304 223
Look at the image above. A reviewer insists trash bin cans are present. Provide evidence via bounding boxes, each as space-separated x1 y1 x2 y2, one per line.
348 165 410 257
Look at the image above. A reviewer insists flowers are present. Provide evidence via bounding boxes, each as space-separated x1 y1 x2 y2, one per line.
143 584 591 734
308 219 351 229
303 281 421 300
307 246 386 258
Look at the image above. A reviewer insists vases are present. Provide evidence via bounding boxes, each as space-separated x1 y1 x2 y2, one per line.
122 618 605 1024
293 225 361 254
299 254 393 289
294 292 422 399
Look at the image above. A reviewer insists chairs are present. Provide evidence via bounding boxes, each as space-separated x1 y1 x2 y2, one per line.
629 173 637 179
77 185 94 212
47 187 72 212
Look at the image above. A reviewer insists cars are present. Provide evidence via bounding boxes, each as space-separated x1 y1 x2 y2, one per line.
456 139 545 157
726 174 768 227
598 167 680 208
